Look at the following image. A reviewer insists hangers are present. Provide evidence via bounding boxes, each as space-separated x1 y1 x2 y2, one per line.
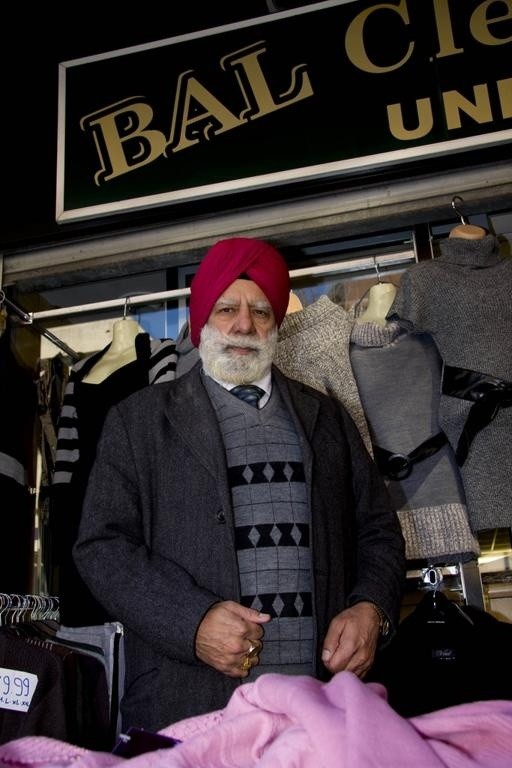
0 590 63 650
418 569 476 643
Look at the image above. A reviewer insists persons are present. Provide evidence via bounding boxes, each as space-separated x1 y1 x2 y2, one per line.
70 238 407 736
58 292 177 628
384 192 512 531
274 288 373 460
346 252 479 569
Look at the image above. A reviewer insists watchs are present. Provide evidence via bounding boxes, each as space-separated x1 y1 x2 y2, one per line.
369 602 391 642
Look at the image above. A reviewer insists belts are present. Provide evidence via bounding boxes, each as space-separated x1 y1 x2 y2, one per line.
371 428 450 483
439 364 512 469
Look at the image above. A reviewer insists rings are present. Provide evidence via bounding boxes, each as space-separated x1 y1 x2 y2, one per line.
239 653 252 672
246 639 260 657
